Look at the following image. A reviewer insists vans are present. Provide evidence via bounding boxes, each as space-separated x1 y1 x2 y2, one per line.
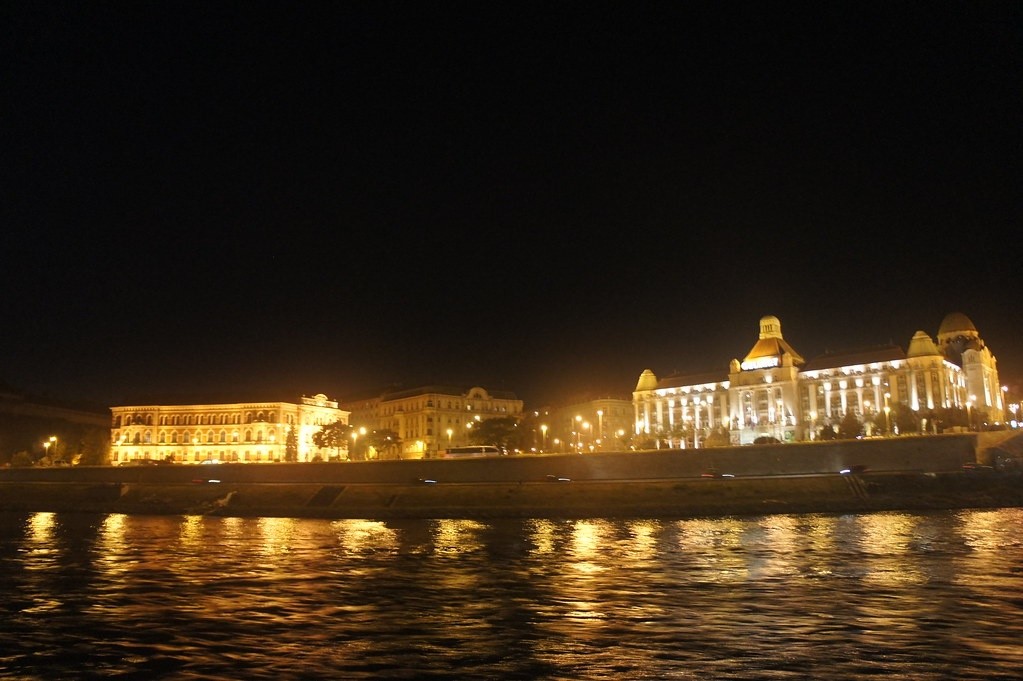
443 446 500 458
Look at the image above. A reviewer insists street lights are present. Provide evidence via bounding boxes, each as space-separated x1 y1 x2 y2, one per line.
50 438 57 448
542 426 546 446
44 443 50 454
598 411 603 438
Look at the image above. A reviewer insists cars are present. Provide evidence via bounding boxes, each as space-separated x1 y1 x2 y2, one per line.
119 462 137 466
54 460 72 466
